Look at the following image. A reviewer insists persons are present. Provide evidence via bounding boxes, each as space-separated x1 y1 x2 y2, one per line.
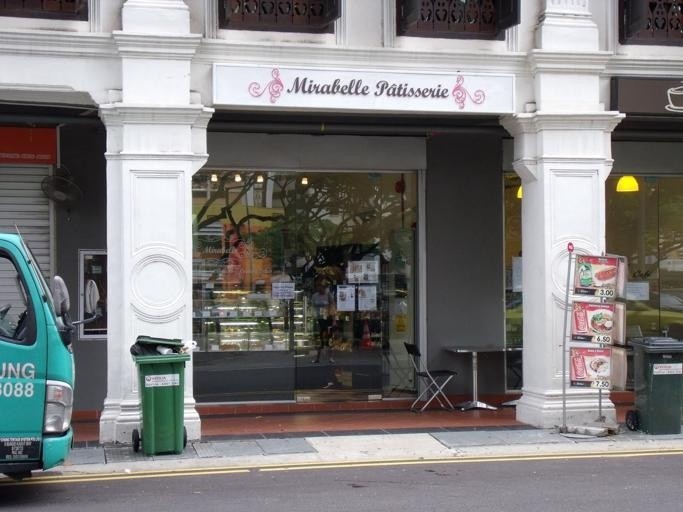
311 280 335 347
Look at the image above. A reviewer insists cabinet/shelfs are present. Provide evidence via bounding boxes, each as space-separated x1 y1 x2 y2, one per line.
292 289 309 394
308 243 390 391
192 279 297 406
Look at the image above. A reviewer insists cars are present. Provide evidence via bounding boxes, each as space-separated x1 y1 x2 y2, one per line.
506 286 682 341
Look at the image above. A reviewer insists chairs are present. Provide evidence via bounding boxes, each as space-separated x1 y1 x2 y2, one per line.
404 340 458 415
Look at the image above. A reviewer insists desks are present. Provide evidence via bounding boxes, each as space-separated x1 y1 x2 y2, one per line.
440 344 523 412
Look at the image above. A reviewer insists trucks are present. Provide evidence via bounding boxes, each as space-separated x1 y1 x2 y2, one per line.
1 222 105 482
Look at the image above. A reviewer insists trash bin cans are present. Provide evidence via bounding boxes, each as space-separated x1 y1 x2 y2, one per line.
626 338 683 434
132 335 191 454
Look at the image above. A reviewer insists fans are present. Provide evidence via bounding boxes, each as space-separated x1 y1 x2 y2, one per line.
39 166 83 210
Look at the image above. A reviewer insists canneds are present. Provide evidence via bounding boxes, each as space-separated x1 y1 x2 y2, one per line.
578 262 592 286
572 355 588 379
574 308 589 333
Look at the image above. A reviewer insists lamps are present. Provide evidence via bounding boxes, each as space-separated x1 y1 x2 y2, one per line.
615 173 639 193
